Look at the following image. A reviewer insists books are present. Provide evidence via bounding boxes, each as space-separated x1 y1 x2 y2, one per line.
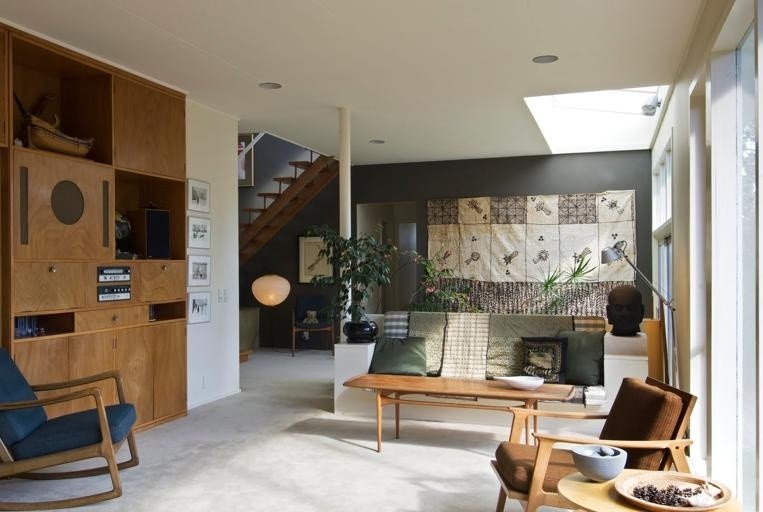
14 314 37 339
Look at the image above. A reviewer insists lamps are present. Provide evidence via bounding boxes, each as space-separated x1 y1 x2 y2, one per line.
601 241 679 388
252 274 291 307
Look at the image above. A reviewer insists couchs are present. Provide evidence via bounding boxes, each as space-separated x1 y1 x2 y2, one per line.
334 310 648 432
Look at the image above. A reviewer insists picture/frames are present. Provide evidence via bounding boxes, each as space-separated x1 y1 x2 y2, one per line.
187 215 211 250
297 235 335 286
238 133 254 187
188 254 212 287
187 177 210 216
188 291 212 324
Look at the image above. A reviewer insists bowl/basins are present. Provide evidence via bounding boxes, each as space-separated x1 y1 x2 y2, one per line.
571 444 628 483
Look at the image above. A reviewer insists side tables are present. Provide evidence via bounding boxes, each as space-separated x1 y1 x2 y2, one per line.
557 468 740 512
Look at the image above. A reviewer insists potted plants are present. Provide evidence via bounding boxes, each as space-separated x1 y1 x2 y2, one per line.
305 224 398 343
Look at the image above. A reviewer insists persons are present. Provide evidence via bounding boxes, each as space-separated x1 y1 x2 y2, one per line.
605 285 644 337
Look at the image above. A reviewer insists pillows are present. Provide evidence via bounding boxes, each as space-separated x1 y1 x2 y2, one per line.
369 336 426 376
521 336 568 384
557 330 606 385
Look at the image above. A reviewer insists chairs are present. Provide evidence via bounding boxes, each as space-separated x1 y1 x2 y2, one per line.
292 295 334 356
490 374 698 512
0 341 140 511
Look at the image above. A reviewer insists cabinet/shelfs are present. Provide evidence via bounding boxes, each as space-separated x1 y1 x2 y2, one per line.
0 23 188 432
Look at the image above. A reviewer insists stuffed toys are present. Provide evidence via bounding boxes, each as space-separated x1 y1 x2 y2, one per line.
302 310 319 325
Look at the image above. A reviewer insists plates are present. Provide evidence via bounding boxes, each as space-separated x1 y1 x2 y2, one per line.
617 469 732 511
